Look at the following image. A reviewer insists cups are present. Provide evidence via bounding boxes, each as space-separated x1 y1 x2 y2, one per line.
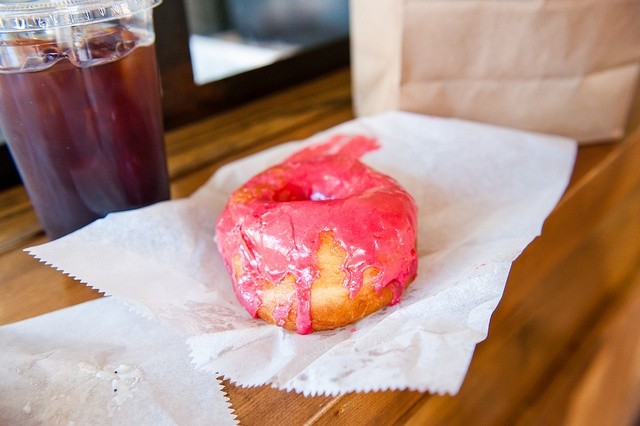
0 0 171 240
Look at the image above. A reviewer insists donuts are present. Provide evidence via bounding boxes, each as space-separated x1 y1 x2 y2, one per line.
213 158 418 331
